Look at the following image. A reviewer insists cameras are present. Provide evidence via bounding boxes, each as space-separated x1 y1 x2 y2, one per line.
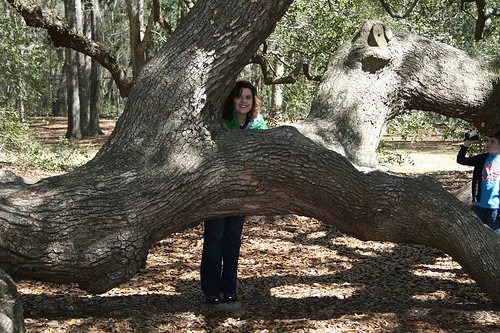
465 130 479 141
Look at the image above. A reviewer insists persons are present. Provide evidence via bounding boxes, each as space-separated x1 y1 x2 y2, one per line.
457 132 500 234
200 80 268 303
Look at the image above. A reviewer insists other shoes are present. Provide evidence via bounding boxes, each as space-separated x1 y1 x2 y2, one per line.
206 296 221 304
457 268 468 276
224 293 237 301
